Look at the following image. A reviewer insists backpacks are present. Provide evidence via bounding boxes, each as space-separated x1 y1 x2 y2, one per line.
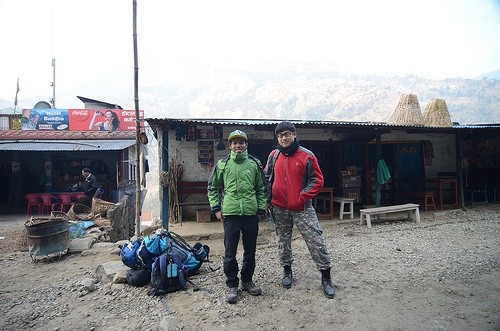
120 230 210 296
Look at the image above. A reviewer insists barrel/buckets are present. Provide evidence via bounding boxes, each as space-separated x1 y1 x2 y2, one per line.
24 220 69 255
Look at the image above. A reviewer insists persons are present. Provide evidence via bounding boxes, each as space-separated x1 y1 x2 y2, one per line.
88 110 121 131
263 122 335 298
206 129 269 305
81 168 102 209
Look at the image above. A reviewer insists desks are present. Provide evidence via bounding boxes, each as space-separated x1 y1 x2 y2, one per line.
315 187 336 220
421 178 459 210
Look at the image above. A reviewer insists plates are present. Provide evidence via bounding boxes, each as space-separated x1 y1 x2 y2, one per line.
57 124 68 130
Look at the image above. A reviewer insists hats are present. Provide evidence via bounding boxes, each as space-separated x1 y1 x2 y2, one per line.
228 130 248 142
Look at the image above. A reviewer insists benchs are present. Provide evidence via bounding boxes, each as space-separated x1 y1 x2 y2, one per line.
442 188 488 208
405 191 435 211
314 196 355 219
359 203 420 228
177 181 209 225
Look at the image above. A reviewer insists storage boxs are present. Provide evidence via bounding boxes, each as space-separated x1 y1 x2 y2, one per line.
195 209 211 223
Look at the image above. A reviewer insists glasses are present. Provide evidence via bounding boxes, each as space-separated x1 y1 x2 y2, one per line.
277 132 295 138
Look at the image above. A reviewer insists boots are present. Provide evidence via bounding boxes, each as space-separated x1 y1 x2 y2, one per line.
282 266 292 288
321 268 334 299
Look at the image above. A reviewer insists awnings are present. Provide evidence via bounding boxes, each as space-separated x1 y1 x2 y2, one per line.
0 140 136 152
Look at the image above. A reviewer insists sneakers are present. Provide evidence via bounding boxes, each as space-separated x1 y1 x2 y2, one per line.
241 280 262 295
227 286 237 303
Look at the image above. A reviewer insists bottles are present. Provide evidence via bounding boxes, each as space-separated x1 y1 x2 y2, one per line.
96 110 107 118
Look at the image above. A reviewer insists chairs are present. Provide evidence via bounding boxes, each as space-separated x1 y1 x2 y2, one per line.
26 192 87 215
